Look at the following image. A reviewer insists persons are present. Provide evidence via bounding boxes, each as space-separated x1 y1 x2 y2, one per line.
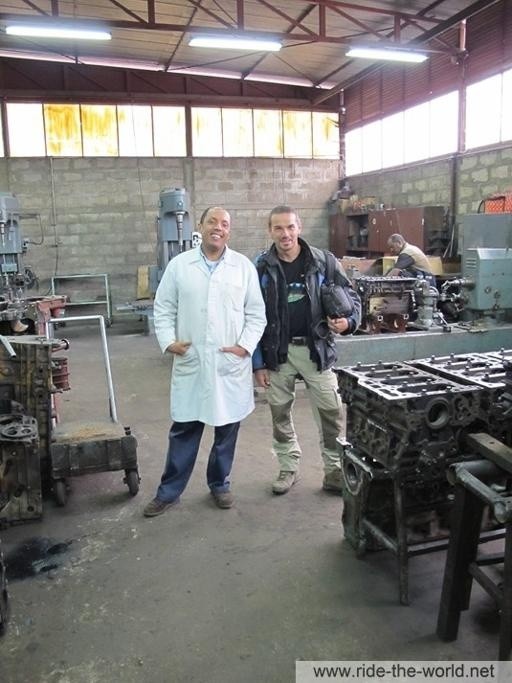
143 206 268 516
252 206 361 494
384 234 437 288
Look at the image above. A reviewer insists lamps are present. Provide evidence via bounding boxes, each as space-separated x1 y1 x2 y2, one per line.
0 12 430 62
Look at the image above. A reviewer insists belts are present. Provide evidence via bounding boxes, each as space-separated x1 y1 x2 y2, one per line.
288 337 308 348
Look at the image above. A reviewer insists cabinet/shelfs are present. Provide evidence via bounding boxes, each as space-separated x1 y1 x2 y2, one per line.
329 205 447 260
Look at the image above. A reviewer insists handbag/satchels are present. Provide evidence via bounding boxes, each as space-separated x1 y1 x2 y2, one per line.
319 280 356 321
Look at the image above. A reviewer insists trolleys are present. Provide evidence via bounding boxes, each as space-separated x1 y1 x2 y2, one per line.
45 313 142 508
51 272 114 330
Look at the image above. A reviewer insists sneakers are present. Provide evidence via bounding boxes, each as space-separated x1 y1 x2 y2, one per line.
323 468 346 494
143 493 181 517
209 486 236 509
271 468 302 496
